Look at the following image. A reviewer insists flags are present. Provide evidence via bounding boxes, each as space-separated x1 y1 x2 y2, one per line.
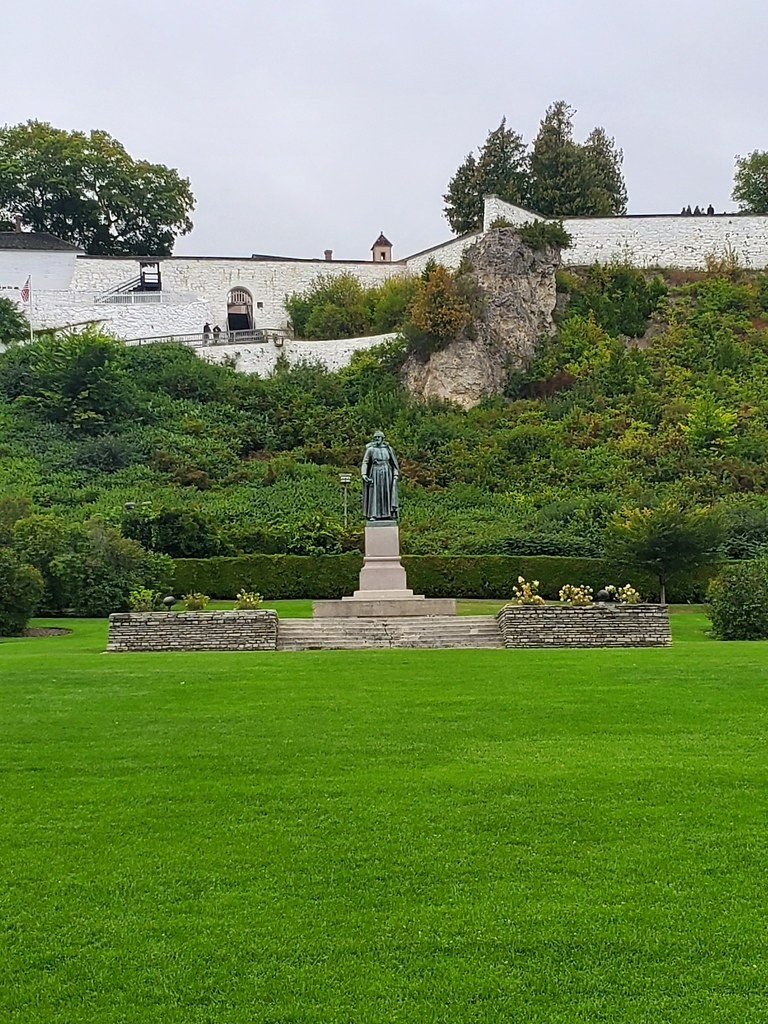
22 278 29 302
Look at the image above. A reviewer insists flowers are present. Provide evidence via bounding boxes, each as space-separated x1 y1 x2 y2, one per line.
181 589 210 611
605 584 642 605
558 584 594 605
512 575 546 605
232 588 264 611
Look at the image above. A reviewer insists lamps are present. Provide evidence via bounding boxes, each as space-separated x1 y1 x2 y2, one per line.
163 596 176 610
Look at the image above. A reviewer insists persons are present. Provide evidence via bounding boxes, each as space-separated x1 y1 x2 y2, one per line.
360 431 399 521
203 322 221 345
681 204 714 215
